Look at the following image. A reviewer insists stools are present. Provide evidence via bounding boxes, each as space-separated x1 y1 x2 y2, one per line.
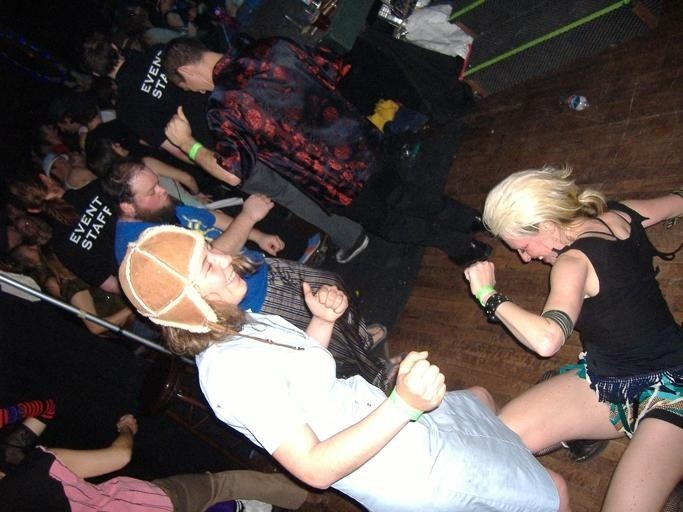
141 363 267 472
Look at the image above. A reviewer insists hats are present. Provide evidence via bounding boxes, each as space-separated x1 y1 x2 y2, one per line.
42 152 69 178
118 224 217 335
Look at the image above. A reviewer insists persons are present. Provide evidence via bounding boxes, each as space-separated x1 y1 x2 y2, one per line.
1 1 489 512
464 169 683 512
117 223 569 512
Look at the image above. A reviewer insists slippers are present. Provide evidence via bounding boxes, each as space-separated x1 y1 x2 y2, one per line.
383 352 407 385
366 323 387 351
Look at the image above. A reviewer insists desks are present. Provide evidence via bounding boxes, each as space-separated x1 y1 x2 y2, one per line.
300 1 376 52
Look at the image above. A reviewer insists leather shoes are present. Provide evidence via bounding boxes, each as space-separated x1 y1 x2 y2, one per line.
463 213 483 235
447 238 491 267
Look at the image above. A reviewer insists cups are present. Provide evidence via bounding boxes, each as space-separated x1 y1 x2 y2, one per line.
307 12 331 32
376 5 404 28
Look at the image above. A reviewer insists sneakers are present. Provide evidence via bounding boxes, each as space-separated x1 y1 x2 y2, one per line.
336 232 369 263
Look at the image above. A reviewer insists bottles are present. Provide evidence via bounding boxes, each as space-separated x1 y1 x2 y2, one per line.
556 92 590 112
213 9 241 30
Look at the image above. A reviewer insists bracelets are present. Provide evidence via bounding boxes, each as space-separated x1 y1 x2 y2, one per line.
476 286 494 303
484 293 509 323
